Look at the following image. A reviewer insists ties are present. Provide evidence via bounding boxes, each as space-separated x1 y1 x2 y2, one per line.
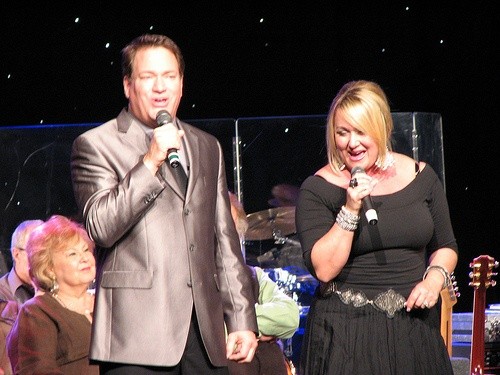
165 158 189 196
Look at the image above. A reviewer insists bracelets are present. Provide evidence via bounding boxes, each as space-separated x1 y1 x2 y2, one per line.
336 205 360 230
422 264 448 291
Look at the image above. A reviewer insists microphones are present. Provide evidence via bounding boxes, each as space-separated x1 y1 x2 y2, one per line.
156 110 179 168
351 166 378 225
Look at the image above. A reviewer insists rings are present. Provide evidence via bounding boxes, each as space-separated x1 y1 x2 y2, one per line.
423 300 429 307
349 178 357 187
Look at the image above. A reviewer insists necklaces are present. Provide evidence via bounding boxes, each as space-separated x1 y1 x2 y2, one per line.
57 295 94 314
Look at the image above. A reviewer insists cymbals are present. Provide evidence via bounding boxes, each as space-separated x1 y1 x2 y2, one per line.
243 205 296 240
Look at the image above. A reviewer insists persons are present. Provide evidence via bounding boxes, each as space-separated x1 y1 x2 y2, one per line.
296 80 458 375
211 188 301 375
72 35 260 375
7 215 101 375
0 220 45 375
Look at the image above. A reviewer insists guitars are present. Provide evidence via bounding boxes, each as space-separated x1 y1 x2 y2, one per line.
436 254 499 375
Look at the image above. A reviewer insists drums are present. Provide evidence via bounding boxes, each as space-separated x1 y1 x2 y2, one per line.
263 269 316 320
